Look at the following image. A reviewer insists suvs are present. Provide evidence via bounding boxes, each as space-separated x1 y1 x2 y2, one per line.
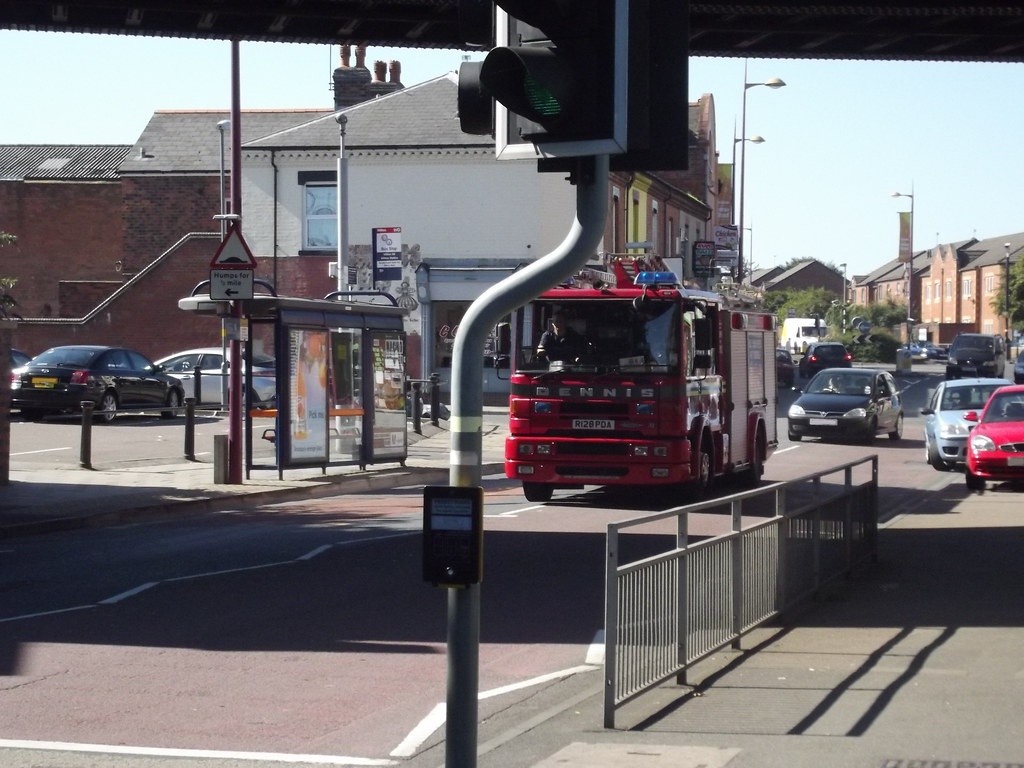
799 342 852 379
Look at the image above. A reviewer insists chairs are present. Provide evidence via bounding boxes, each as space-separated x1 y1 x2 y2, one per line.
211 357 220 369
1006 403 1023 417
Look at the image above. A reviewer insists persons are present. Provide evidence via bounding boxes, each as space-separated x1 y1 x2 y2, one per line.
537 311 585 365
825 377 845 394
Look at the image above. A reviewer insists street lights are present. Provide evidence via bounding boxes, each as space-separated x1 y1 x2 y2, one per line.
731 136 765 283
891 192 913 318
839 263 846 305
739 78 785 285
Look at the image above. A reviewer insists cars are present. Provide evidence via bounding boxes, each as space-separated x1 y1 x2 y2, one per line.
918 379 1022 471
962 384 1024 489
10 345 185 423
777 349 798 384
1014 350 1024 382
900 341 947 360
787 368 905 443
143 347 276 414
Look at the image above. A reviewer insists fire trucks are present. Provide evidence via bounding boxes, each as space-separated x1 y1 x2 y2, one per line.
494 245 778 503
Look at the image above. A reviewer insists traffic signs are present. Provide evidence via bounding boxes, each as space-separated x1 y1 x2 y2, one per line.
210 270 254 301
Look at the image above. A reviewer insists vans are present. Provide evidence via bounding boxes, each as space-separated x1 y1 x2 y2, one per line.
946 333 1005 380
782 318 828 354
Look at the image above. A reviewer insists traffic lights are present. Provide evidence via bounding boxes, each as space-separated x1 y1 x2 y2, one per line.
480 0 627 159
458 0 492 136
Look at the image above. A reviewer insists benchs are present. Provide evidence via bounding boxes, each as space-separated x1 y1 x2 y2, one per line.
263 427 362 443
